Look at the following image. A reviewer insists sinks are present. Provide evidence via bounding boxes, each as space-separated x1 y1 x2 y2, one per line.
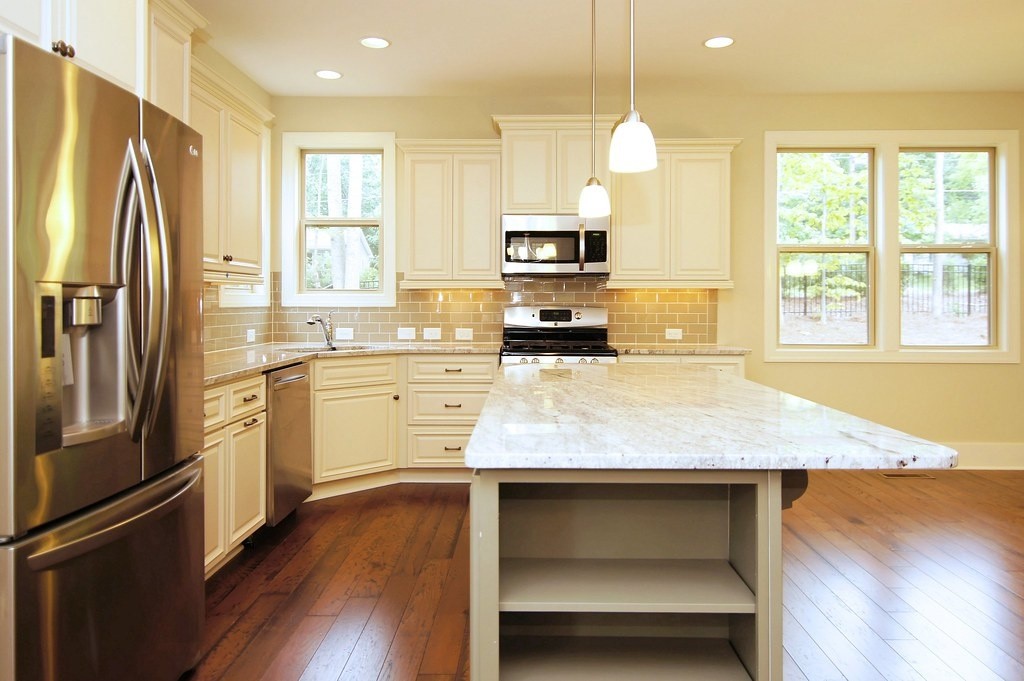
276 345 390 353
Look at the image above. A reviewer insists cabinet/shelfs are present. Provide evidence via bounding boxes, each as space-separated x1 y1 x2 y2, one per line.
313 354 402 500
492 115 624 215
393 138 505 289
204 373 268 580
402 354 499 483
0 0 149 100
607 137 745 289
192 54 276 285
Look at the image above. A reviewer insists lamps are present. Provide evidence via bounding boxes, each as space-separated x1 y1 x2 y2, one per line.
608 0 658 174
578 0 612 218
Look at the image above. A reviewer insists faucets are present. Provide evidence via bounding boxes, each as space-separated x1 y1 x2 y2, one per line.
307 310 335 346
